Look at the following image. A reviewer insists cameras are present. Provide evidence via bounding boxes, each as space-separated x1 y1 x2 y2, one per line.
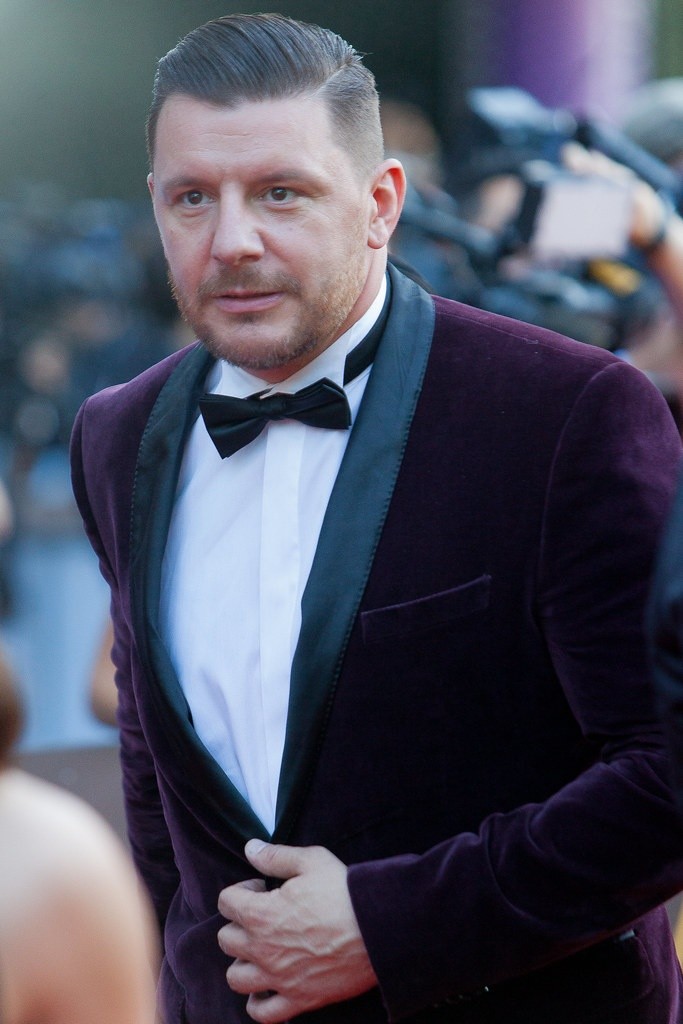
387 206 610 346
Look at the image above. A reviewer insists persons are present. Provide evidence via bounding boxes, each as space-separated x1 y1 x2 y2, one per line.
70 13 683 1024
605 74 683 417
0 626 160 1024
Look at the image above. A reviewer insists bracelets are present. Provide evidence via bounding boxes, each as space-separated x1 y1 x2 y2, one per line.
636 189 676 256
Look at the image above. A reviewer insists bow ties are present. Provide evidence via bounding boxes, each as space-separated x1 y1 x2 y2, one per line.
197 376 351 457
